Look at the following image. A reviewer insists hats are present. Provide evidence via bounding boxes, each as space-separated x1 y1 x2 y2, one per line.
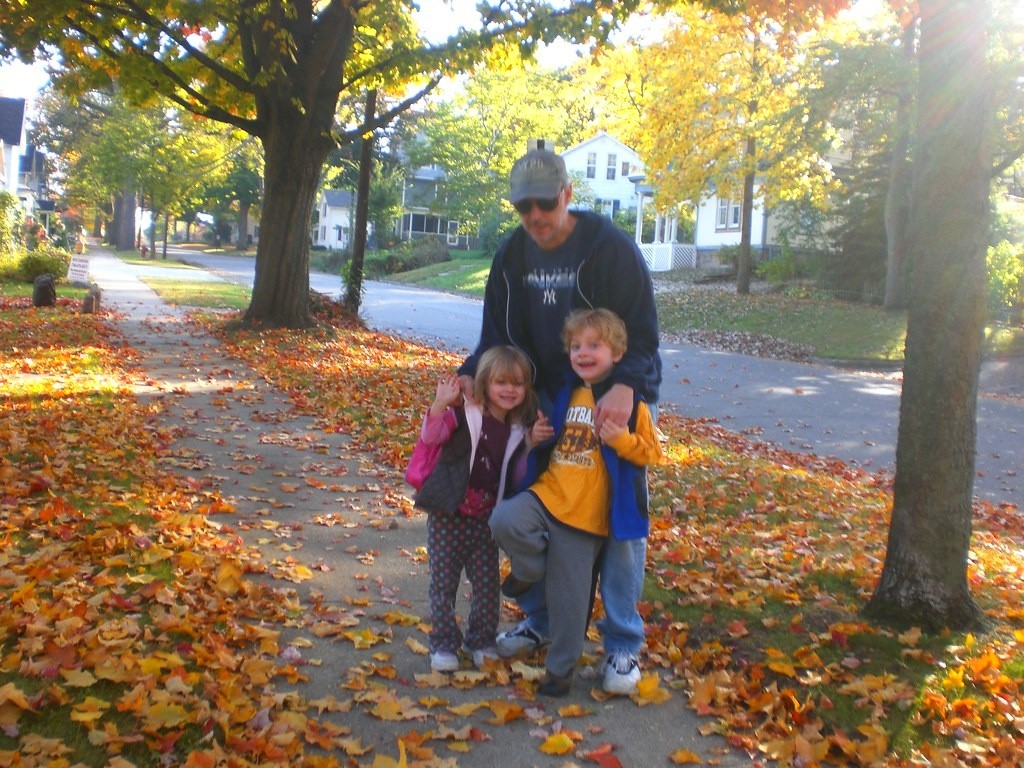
510 150 568 205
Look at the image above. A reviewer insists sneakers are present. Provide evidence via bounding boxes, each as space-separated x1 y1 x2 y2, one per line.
601 649 641 695
430 650 459 673
461 641 499 669
496 619 552 657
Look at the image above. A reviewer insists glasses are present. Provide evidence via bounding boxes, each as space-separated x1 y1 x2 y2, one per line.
513 186 564 214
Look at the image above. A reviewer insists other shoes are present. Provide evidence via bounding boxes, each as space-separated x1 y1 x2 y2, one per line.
502 572 534 598
540 667 573 698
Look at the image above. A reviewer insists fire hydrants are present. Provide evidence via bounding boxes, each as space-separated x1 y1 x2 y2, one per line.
141 245 148 258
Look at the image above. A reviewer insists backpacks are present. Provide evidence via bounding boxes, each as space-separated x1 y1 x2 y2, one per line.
405 406 458 492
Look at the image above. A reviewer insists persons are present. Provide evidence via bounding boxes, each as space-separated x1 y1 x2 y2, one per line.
454 149 664 695
405 344 544 672
489 307 662 698
